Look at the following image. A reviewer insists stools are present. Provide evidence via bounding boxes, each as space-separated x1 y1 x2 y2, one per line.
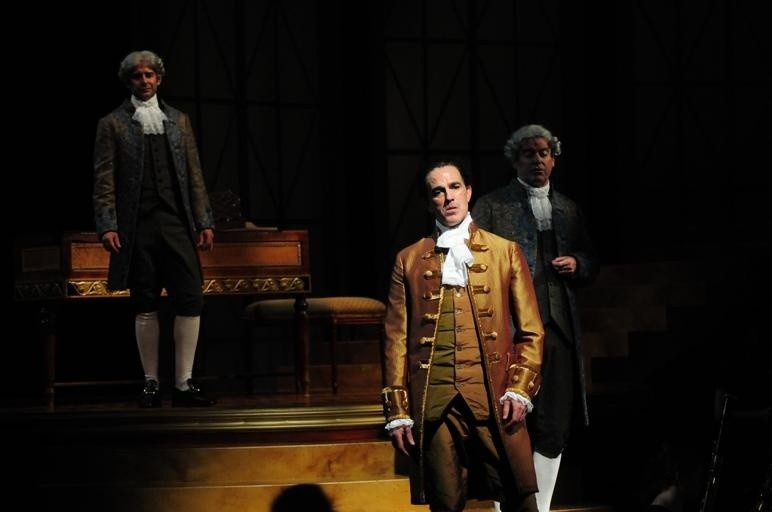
243 289 391 399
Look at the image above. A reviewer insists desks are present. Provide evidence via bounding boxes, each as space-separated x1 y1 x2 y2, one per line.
13 231 316 414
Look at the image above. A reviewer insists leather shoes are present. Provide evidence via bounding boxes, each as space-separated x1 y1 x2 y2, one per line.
171 379 216 408
138 380 162 408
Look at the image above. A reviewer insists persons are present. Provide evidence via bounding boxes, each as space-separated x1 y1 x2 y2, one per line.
93 50 218 409
382 157 545 512
470 124 602 512
